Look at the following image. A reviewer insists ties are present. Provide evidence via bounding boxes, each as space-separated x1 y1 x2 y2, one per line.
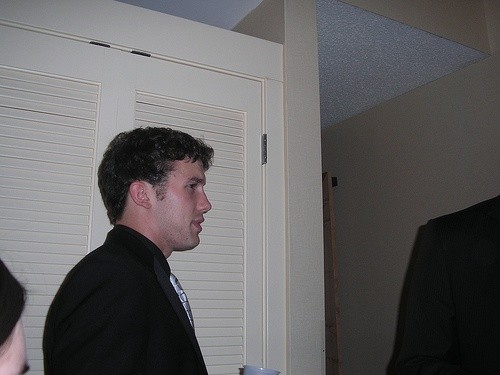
169 273 194 330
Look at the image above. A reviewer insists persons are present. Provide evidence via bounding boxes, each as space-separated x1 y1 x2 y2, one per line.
0 260 30 375
388 193 500 375
42 126 213 375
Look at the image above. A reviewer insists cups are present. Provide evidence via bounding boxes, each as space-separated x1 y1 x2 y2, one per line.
243 365 280 375
